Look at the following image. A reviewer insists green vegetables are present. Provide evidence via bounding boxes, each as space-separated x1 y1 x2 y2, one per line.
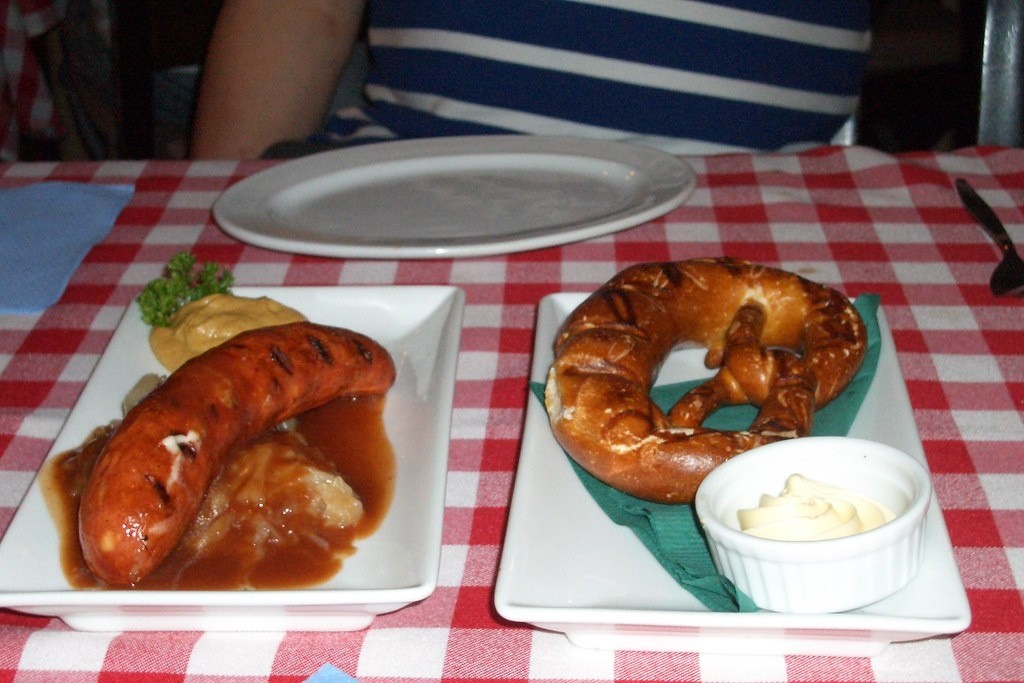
135 251 236 327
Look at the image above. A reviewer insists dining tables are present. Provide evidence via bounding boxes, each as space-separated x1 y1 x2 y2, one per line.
0 146 1024 683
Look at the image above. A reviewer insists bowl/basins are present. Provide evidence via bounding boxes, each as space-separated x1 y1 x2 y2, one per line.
694 434 932 614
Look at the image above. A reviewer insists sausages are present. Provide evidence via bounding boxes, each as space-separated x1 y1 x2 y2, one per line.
77 320 398 584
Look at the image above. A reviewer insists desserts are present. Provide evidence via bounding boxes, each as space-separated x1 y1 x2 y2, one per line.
731 474 900 542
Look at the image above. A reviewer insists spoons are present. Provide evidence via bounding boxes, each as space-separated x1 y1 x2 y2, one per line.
954 176 1024 297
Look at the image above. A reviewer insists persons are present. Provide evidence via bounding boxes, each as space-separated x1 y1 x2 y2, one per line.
190 0 880 162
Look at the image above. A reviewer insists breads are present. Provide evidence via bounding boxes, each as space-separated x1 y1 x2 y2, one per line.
542 253 866 505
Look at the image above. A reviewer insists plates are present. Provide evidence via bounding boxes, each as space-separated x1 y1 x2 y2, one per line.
0 284 465 628
212 131 697 258
494 290 971 639
